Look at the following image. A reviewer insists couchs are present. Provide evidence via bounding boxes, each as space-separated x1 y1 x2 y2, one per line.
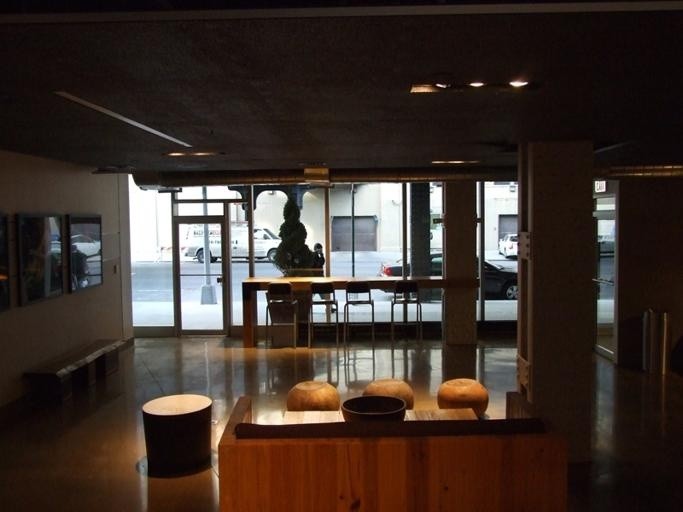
217 396 568 512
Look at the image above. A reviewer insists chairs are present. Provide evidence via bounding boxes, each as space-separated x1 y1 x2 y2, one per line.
264 279 424 349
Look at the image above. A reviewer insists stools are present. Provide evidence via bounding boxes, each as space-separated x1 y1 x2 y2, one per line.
362 378 416 409
141 392 211 478
437 379 489 413
286 380 341 410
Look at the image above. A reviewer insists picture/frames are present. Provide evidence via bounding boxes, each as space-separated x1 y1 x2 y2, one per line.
0 213 104 312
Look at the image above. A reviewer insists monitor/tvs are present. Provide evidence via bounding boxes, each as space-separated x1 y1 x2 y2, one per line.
0 214 10 312
15 213 62 307
66 214 103 293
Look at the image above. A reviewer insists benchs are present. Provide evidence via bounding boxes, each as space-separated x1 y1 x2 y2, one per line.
25 336 132 405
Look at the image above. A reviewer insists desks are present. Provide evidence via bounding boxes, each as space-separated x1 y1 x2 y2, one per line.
242 276 481 349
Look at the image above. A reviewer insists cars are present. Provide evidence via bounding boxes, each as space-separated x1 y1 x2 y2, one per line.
379 248 518 301
499 233 519 259
52 233 101 257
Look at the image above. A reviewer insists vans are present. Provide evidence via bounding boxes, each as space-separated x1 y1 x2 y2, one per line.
187 226 282 263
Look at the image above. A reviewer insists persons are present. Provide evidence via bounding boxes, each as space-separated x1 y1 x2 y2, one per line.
311 243 336 313
